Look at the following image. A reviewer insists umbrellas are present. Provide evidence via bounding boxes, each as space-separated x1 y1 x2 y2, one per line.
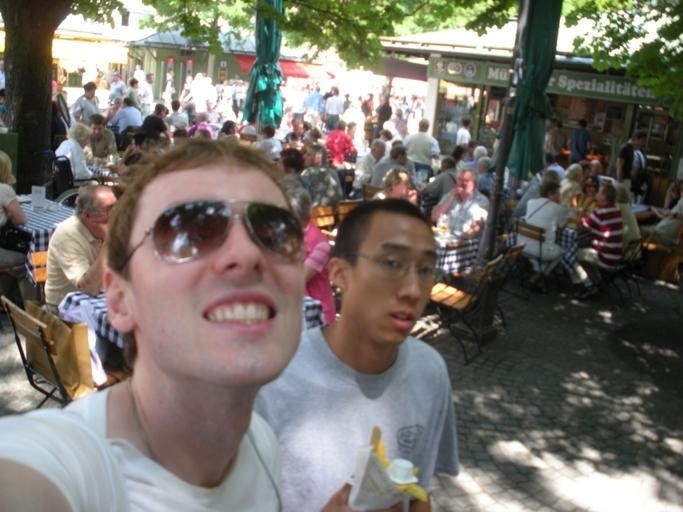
244 0 287 142
450 0 562 344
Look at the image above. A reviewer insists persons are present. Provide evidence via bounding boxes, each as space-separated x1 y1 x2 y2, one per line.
0 135 309 512
252 194 460 511
0 68 683 329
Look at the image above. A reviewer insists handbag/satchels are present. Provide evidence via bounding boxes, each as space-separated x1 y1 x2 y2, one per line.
0 220 31 253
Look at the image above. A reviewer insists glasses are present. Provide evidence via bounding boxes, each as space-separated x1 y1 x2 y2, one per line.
347 250 444 282
119 199 305 264
